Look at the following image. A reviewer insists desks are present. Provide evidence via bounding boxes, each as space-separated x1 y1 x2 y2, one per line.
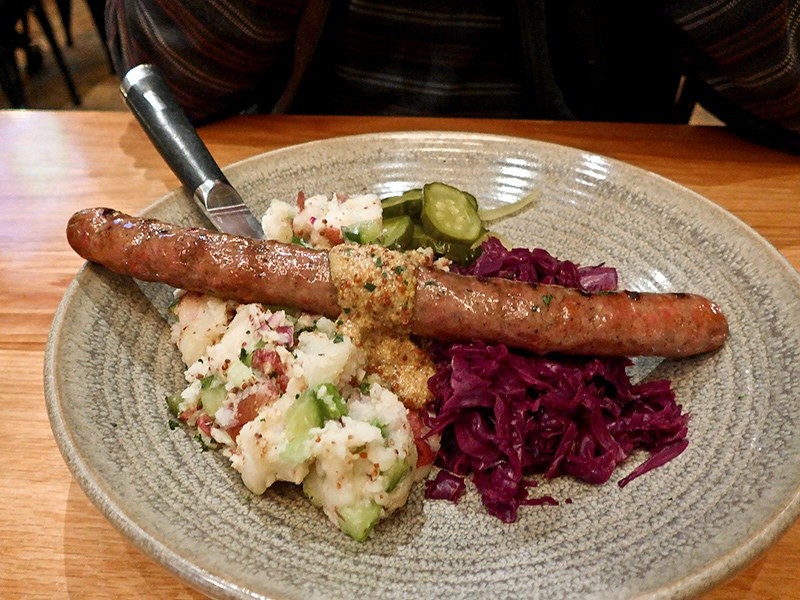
0 105 799 600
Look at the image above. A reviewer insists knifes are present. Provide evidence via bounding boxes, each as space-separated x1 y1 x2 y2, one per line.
120 63 265 241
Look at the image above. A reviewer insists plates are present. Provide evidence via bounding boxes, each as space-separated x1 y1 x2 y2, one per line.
42 130 800 600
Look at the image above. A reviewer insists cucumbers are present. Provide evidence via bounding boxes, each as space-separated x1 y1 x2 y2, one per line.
365 182 491 263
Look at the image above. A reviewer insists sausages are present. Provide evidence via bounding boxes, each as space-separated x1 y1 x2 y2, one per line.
66 209 729 356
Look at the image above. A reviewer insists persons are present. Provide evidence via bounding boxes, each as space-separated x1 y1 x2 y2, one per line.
100 0 799 154
